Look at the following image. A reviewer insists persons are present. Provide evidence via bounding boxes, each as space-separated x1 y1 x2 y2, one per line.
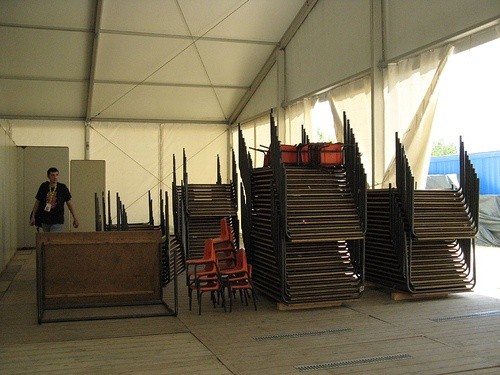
30 167 80 233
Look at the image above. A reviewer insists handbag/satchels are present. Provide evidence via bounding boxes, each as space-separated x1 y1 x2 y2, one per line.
30 210 42 227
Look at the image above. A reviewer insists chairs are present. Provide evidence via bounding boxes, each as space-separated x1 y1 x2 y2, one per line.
249 124 345 166
186 217 257 315
